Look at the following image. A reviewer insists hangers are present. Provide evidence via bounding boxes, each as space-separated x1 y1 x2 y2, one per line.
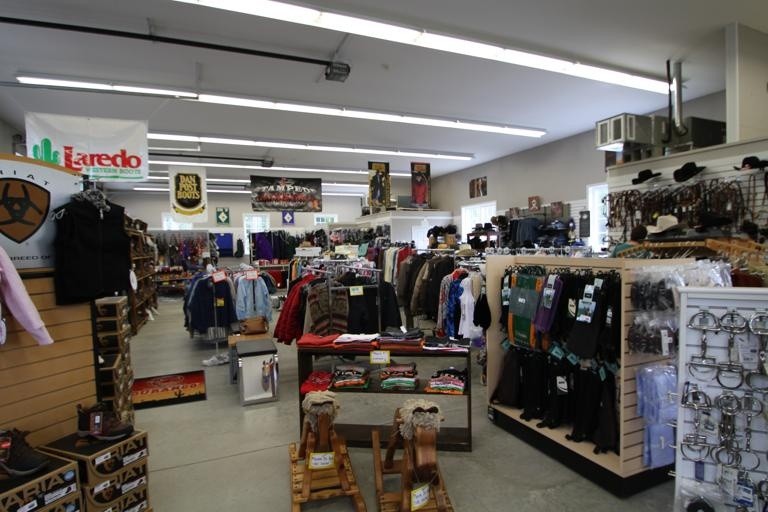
381 237 417 254
616 238 767 283
207 262 256 280
452 245 590 273
408 246 457 266
302 256 378 288
47 175 113 223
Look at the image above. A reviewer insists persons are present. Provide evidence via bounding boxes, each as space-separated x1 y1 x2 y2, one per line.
412 173 426 204
370 170 384 206
612 226 649 259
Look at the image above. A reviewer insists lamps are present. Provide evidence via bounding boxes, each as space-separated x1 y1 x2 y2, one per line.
18 73 548 195
158 0 673 94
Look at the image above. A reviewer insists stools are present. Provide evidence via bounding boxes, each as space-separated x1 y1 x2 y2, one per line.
224 329 272 385
235 339 282 409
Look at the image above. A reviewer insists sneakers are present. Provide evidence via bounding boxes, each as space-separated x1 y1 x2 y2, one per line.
77 401 135 440
1 428 50 474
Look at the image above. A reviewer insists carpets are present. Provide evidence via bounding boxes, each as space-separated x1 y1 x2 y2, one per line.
131 369 207 412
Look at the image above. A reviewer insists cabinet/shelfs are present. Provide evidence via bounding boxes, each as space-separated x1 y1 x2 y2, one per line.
293 333 475 458
122 226 159 335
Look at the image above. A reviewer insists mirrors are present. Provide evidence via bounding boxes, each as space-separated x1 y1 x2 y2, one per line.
240 353 274 402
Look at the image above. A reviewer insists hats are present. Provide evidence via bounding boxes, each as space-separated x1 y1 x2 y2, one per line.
472 223 496 234
733 155 767 170
645 214 684 236
674 162 706 182
695 211 734 234
631 169 661 184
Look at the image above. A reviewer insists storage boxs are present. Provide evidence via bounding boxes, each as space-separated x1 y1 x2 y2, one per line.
0 425 155 512
95 295 138 435
